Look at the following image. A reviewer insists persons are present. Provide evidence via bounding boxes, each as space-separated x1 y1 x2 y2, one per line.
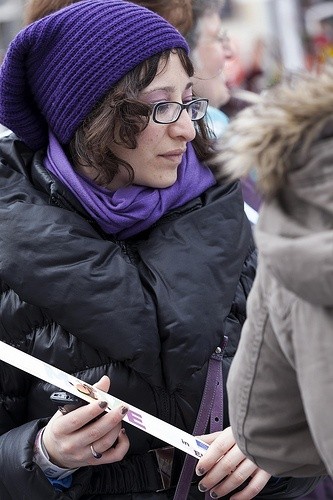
214 28 270 118
130 0 333 482
0 0 320 500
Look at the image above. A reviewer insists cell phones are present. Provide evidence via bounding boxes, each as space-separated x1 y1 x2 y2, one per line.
50 391 108 430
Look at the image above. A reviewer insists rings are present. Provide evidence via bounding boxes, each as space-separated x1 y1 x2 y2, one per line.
90 443 102 458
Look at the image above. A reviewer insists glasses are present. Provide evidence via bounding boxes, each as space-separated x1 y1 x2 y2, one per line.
144 97 209 125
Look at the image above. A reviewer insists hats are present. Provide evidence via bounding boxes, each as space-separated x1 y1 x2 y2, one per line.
0 0 189 145
